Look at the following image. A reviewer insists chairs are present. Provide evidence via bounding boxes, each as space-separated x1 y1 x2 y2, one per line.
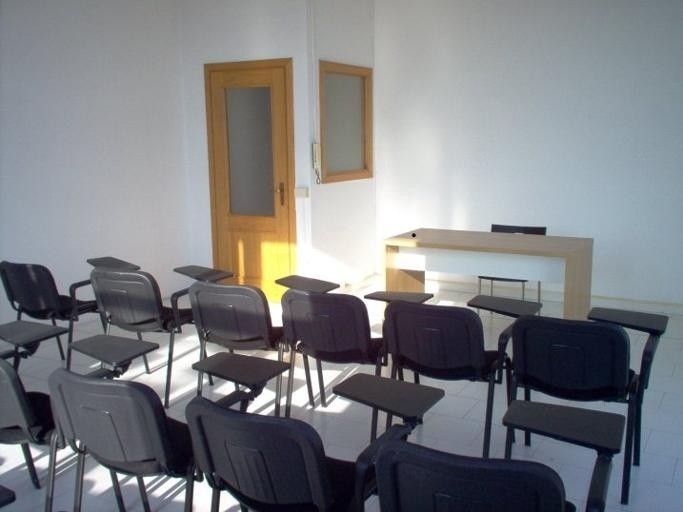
376 439 614 512
382 299 516 458
478 224 546 316
504 314 660 506
48 367 266 512
281 289 404 444
0 260 107 373
90 266 214 409
185 395 424 512
188 281 315 417
0 357 132 512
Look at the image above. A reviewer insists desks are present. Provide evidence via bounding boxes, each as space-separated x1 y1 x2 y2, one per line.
383 228 594 321
